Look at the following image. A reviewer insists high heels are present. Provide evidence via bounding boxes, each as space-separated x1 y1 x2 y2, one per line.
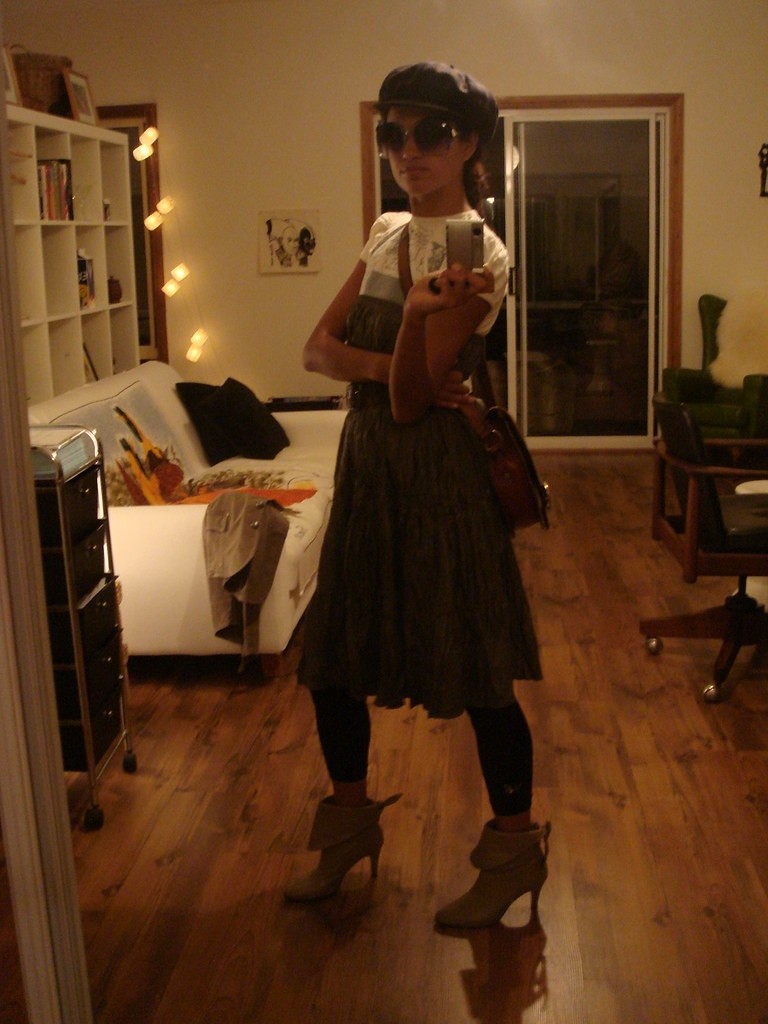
288 789 405 897
434 816 551 927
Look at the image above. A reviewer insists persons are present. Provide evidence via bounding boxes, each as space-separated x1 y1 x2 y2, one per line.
280 61 550 933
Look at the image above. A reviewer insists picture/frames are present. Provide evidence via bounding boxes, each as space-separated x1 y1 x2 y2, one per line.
63 67 102 127
0 46 23 106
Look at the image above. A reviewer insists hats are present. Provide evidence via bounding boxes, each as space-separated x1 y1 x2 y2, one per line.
375 63 498 139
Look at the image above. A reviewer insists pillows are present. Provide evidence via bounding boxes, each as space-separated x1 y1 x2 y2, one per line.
174 381 239 466
198 376 291 464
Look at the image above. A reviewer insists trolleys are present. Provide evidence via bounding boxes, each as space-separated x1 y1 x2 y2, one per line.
30 422 136 831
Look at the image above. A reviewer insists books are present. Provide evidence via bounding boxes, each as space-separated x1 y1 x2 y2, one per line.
38 159 71 221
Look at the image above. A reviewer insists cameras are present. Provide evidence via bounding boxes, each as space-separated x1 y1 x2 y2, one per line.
446 219 484 275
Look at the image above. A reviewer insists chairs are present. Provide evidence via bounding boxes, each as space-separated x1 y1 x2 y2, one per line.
640 391 768 702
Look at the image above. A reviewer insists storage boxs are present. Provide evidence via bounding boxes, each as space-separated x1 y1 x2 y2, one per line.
33 464 122 772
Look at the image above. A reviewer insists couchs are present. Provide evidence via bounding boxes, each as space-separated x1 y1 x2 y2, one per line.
662 293 768 463
28 361 347 657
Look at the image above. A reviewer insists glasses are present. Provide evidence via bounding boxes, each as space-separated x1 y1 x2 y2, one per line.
374 113 467 153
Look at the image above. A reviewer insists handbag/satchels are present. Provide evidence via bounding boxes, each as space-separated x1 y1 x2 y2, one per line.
472 399 551 537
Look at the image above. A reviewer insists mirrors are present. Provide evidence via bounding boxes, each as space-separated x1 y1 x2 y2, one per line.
0 0 768 1024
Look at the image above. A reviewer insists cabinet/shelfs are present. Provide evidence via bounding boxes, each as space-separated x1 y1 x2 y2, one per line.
2 101 139 417
28 424 139 832
